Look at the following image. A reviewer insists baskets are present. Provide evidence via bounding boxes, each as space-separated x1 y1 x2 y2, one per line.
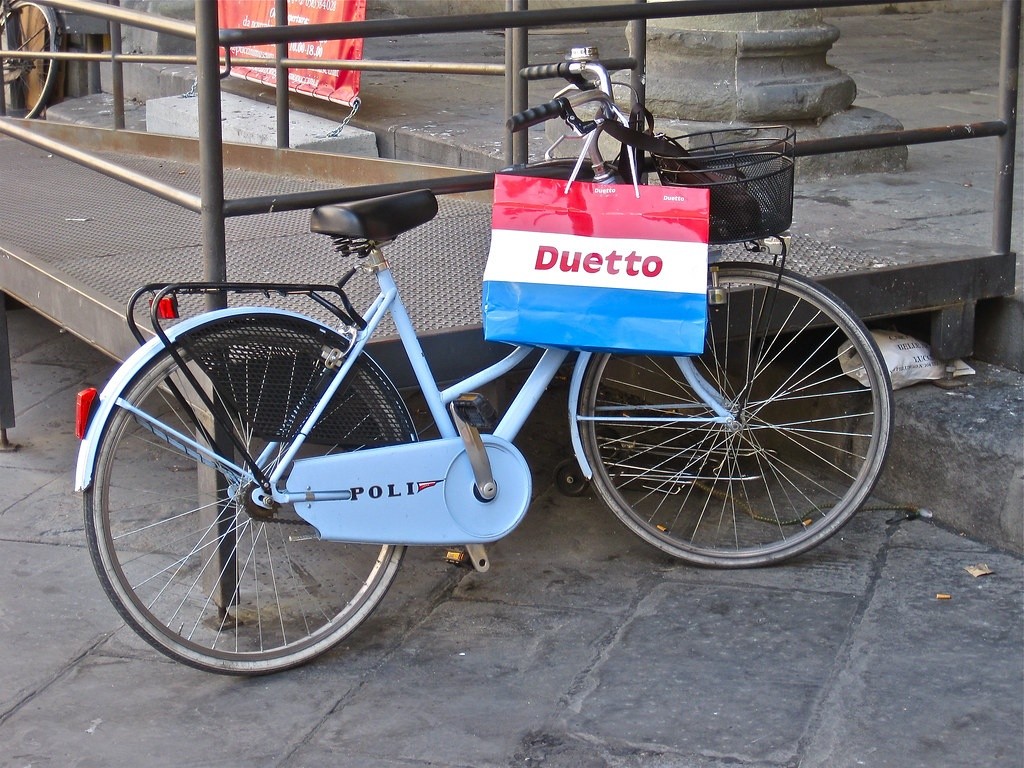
651 124 797 243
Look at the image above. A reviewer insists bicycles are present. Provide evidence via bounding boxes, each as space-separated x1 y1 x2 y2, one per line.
0 0 63 119
74 46 897 677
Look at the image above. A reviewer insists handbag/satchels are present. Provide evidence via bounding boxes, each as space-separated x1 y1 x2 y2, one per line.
482 106 711 355
